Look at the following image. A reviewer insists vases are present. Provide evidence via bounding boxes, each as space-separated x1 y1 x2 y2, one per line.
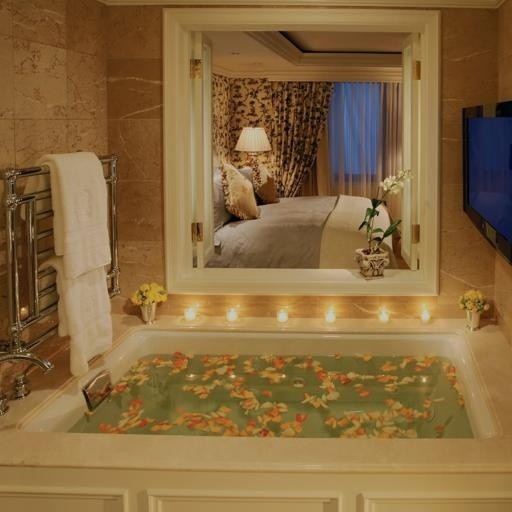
139 302 159 326
463 308 483 332
356 246 392 281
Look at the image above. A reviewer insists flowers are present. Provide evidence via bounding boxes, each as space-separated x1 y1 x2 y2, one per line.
360 163 414 249
128 282 170 307
457 288 492 314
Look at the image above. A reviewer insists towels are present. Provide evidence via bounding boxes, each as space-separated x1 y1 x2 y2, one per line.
17 147 119 380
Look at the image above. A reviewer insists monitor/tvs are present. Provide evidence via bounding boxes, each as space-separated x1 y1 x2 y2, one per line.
462 101 512 264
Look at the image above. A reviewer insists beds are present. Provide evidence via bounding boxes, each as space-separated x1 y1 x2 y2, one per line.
200 156 395 269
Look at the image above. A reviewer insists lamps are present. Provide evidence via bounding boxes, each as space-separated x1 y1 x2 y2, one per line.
234 125 275 164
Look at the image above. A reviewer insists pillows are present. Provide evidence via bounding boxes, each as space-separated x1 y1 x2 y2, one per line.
220 160 263 221
255 163 282 206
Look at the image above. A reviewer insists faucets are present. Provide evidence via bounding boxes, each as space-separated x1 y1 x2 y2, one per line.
0 324 55 415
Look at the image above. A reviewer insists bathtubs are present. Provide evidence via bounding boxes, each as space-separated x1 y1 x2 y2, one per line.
0 315 512 474
